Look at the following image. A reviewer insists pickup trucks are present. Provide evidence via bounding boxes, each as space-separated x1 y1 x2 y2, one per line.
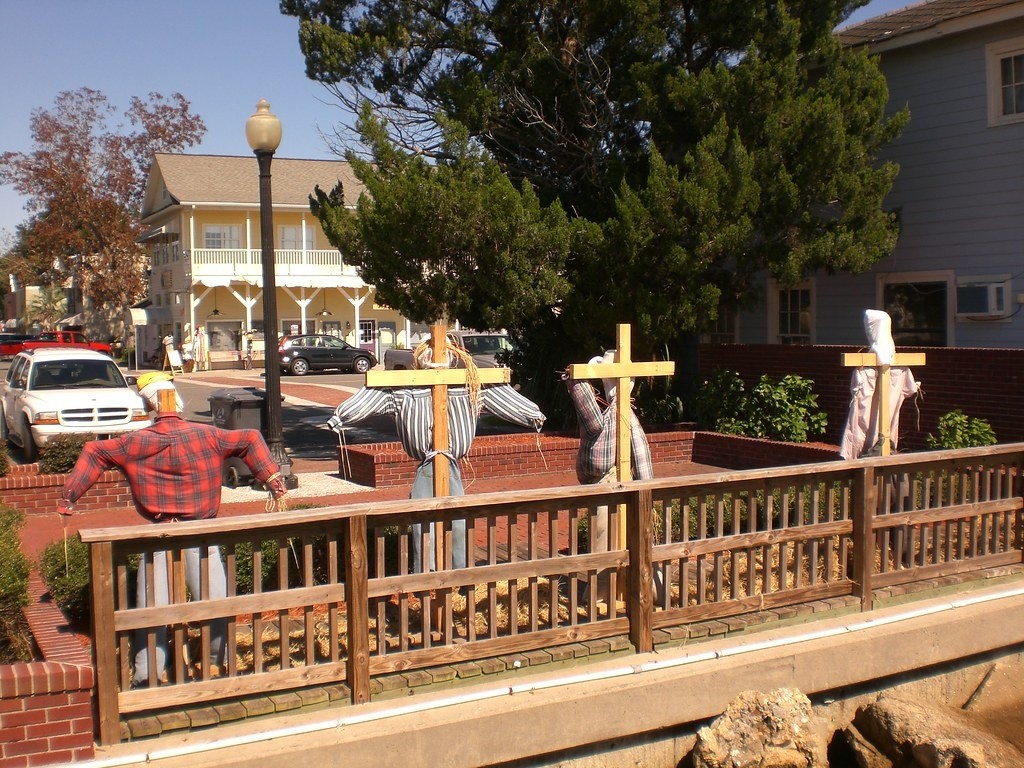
0 331 114 361
385 334 525 370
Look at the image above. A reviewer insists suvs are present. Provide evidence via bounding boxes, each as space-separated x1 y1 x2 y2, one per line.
0 348 150 464
278 334 379 376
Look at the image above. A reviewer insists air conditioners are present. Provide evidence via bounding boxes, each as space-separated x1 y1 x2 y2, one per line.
954 282 1005 319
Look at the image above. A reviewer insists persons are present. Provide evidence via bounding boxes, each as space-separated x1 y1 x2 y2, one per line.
329 331 548 597
58 411 290 693
280 328 291 344
560 351 657 603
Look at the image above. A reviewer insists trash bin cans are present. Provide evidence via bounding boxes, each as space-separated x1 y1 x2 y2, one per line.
128 350 136 370
207 387 285 439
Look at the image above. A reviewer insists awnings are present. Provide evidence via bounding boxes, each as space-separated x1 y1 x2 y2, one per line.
3 318 19 329
54 311 86 326
124 308 175 372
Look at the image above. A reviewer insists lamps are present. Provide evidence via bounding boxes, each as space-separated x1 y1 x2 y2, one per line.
315 288 334 316
206 288 226 319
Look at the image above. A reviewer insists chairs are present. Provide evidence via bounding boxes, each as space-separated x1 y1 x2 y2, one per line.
35 369 57 387
77 370 98 382
299 338 304 346
317 341 326 347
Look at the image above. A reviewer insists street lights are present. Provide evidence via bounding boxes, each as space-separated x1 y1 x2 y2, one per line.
246 98 298 489
